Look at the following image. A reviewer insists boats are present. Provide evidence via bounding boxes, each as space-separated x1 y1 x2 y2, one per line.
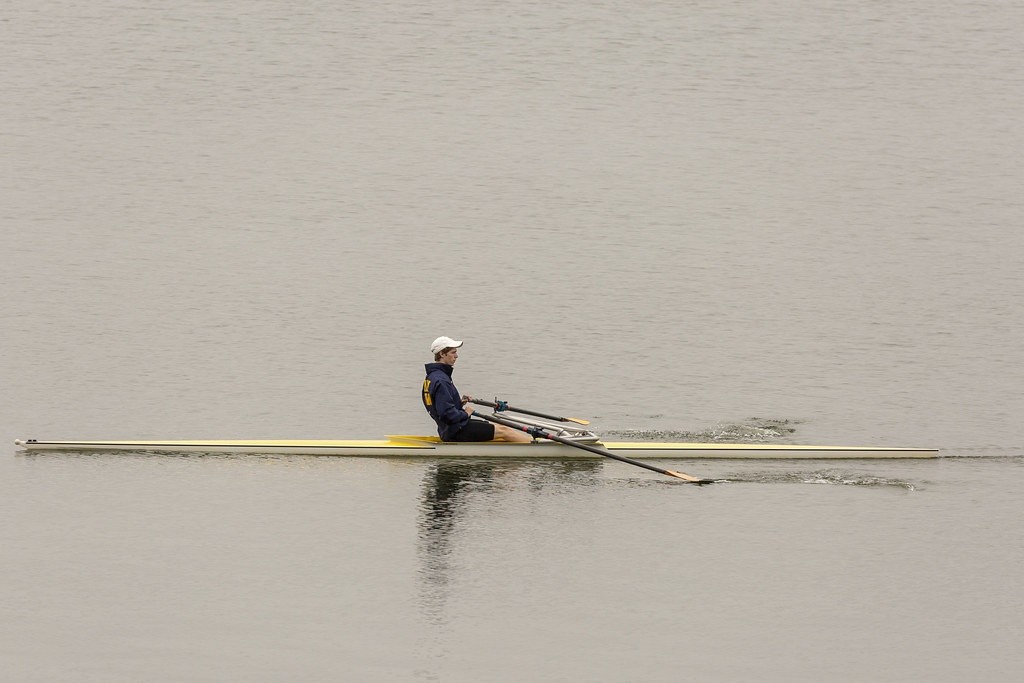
21 434 939 459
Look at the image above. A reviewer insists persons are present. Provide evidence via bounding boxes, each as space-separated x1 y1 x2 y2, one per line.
422 336 553 442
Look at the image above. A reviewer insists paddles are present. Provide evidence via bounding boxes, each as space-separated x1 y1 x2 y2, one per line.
469 395 591 426
472 409 716 485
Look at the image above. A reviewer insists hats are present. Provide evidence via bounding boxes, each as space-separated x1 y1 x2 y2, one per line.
431 337 463 354
